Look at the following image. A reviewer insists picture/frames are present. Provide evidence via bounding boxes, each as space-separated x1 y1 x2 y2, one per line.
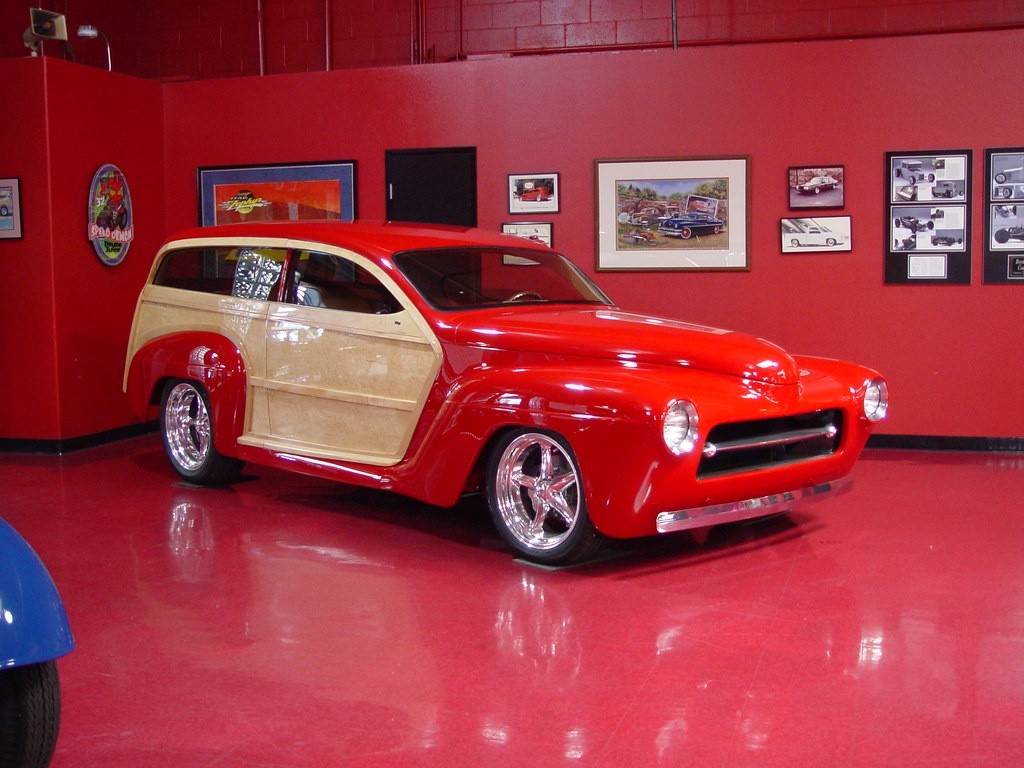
984 145 1024 284
507 172 560 213
780 214 852 253
789 164 845 208
592 154 751 272
0 177 25 240
885 149 972 284
195 158 357 279
501 222 553 266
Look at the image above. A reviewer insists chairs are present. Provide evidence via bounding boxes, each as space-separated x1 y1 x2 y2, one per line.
301 250 368 313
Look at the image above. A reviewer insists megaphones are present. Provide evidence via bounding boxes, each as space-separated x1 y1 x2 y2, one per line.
23 8 68 50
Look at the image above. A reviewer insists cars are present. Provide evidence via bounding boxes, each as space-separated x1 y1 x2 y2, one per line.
995 165 1024 182
994 225 1024 243
0 191 13 215
124 222 891 565
895 159 934 185
519 187 549 202
0 517 75 768
1003 185 1024 199
932 181 958 197
895 234 916 250
933 236 962 246
895 216 934 233
785 226 844 247
796 177 838 195
